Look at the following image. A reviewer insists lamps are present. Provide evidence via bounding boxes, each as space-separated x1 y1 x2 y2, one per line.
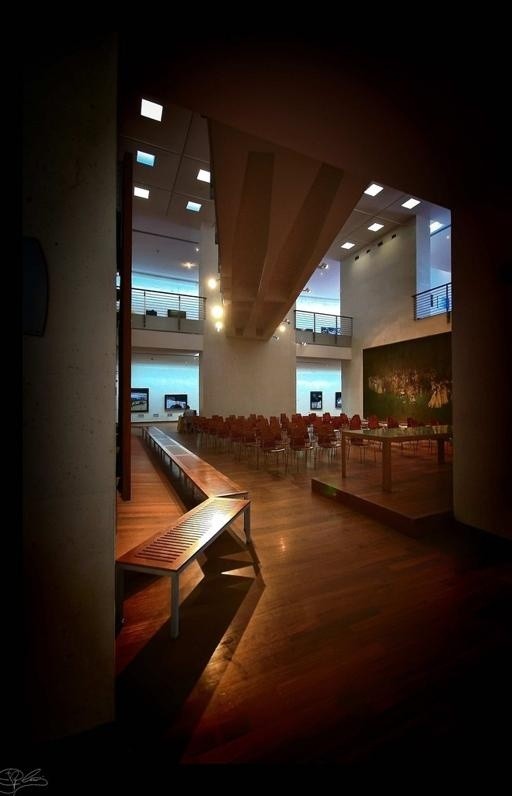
271 262 329 343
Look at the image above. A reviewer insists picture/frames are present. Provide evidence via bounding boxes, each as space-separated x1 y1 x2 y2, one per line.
131 387 149 413
309 391 322 410
335 392 342 409
165 394 188 410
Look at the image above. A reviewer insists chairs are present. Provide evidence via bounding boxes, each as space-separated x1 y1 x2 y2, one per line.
189 412 452 487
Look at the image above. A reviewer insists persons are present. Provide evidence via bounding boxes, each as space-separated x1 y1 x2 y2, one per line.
184 405 195 416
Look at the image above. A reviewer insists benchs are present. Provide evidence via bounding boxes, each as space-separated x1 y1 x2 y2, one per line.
141 423 253 539
116 497 254 642
